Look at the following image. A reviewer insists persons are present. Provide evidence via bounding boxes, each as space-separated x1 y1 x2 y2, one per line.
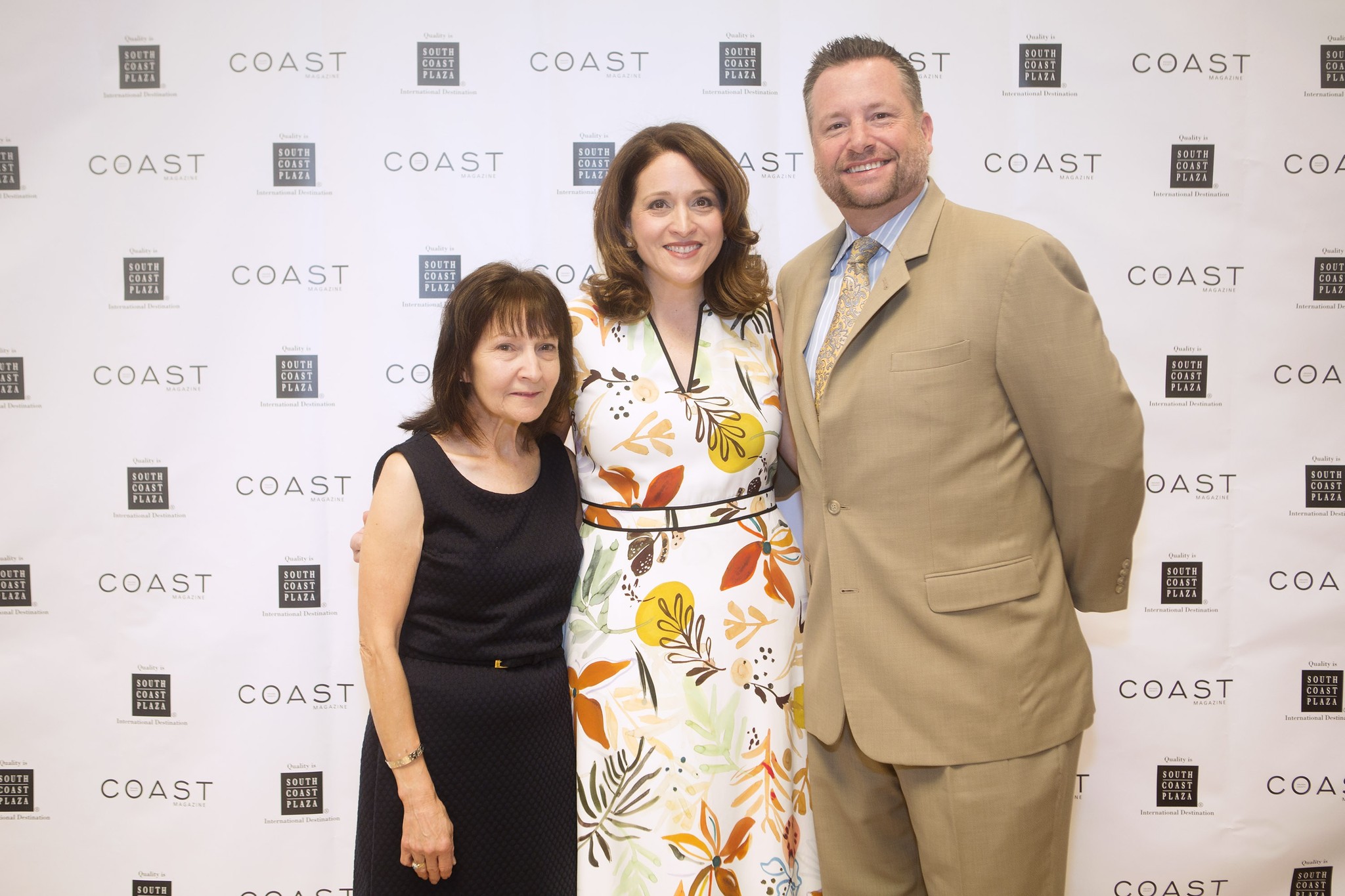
358 260 581 896
350 125 824 896
769 34 1145 896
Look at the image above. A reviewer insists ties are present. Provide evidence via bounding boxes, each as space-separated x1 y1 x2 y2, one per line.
814 236 882 422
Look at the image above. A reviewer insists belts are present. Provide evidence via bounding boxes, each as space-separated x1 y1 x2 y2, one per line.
397 649 559 668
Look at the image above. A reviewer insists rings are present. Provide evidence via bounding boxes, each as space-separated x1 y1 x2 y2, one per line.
411 860 426 870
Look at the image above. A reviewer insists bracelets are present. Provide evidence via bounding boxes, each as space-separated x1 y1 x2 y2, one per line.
385 743 425 769
